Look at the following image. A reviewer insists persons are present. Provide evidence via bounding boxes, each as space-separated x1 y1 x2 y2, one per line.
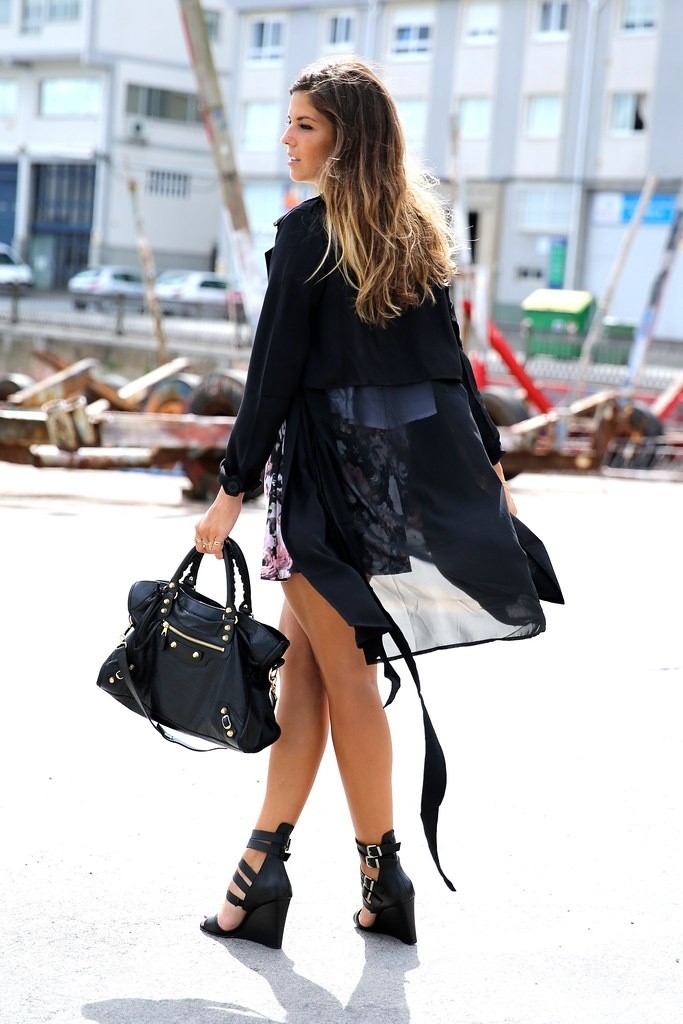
192 56 564 951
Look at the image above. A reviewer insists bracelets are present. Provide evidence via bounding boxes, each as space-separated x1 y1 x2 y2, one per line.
501 479 509 489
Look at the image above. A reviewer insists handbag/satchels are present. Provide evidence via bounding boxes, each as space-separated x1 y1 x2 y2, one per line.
95 536 292 756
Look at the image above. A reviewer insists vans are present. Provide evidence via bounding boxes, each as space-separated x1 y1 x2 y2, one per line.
0 242 35 298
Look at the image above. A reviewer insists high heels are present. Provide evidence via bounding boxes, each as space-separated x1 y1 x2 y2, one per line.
198 821 295 950
352 829 418 946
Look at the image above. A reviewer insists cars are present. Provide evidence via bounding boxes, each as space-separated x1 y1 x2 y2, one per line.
67 267 143 311
152 269 242 316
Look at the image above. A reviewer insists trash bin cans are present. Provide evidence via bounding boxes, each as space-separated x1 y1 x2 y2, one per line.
522 287 596 360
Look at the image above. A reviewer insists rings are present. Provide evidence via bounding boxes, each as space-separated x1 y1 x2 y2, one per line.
212 541 224 546
208 542 213 548
202 540 208 547
195 537 200 543
